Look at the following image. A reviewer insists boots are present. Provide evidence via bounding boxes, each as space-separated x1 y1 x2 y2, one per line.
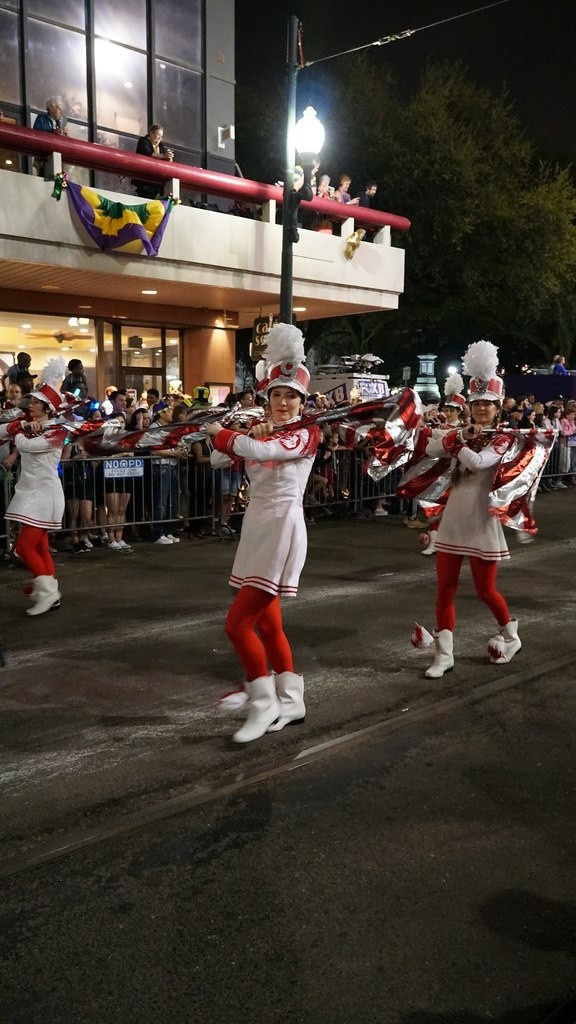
221 674 280 742
410 622 454 679
419 529 440 555
487 616 522 664
21 575 61 616
266 671 306 734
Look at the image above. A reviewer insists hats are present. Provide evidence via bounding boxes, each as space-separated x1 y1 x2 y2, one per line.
17 371 38 383
153 402 171 416
510 405 525 412
263 323 310 406
461 339 503 405
444 374 466 411
20 355 66 414
553 394 566 401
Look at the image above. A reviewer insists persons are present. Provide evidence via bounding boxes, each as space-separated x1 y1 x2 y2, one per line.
131 125 174 200
548 355 569 375
203 360 320 743
296 156 376 242
0 353 269 559
410 375 522 678
0 383 66 615
311 374 576 555
32 96 67 176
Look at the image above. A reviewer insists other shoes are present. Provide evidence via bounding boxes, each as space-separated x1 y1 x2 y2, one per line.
49 531 108 553
534 478 576 494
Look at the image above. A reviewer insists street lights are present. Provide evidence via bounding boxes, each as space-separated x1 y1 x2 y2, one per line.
279 17 326 325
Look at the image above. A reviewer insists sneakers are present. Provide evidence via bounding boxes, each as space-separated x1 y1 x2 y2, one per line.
374 507 388 516
403 516 429 529
166 534 180 543
153 534 173 544
108 540 122 550
117 540 131 549
218 523 236 535
